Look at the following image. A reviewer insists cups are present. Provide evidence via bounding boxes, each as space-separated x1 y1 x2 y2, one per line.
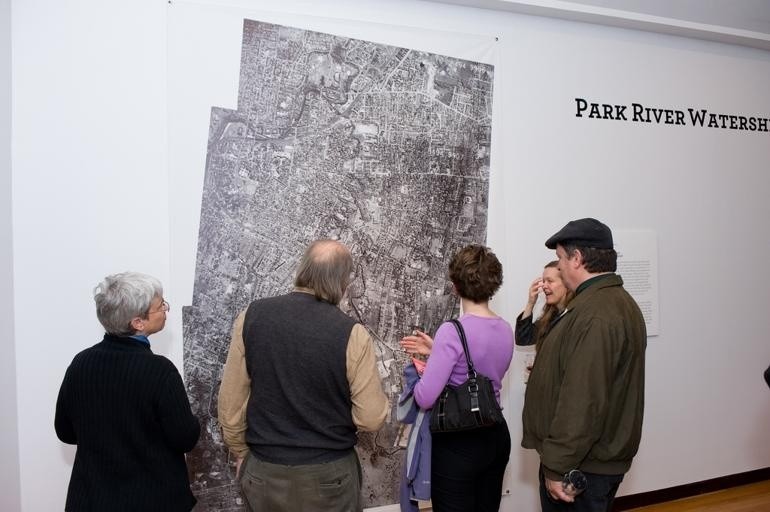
562 469 588 497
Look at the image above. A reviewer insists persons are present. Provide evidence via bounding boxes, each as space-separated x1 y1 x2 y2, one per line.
763 366 770 390
400 244 516 512
217 239 390 512
514 260 570 348
52 273 202 511
518 217 648 511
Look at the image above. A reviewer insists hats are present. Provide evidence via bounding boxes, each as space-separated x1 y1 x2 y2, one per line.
545 218 613 249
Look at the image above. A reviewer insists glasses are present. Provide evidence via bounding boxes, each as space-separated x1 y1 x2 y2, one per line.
146 301 170 314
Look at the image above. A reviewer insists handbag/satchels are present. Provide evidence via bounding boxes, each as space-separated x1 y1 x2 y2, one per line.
430 374 503 434
